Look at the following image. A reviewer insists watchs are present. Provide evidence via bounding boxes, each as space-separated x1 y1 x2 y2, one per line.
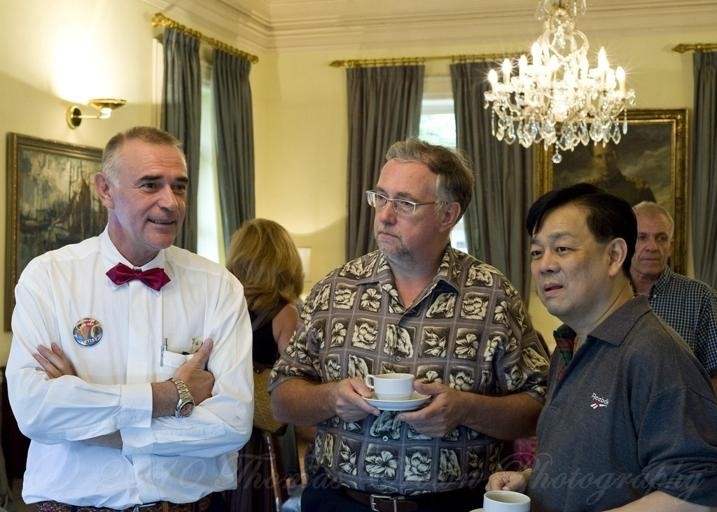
165 376 195 420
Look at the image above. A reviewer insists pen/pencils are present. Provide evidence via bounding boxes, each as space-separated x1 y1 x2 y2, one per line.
159 337 169 367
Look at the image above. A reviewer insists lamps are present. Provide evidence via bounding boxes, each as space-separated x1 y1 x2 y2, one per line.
479 0 636 168
64 98 126 132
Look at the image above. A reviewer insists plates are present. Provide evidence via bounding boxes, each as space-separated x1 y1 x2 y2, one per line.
360 391 433 413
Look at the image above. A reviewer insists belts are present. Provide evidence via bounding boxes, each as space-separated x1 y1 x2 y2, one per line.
342 488 484 512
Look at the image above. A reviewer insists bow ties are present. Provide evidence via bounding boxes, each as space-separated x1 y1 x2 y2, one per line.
106 263 171 291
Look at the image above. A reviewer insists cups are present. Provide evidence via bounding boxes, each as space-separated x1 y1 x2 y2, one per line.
482 489 533 512
365 369 418 400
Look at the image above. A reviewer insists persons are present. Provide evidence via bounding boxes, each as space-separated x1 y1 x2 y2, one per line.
625 199 717 395
267 133 554 512
206 215 307 511
579 138 656 207
4 123 258 512
483 176 716 512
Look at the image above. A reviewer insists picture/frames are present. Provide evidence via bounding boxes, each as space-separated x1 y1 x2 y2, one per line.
4 131 108 334
533 107 692 276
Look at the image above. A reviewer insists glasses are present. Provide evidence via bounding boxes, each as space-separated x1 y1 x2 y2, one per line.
366 189 436 215
